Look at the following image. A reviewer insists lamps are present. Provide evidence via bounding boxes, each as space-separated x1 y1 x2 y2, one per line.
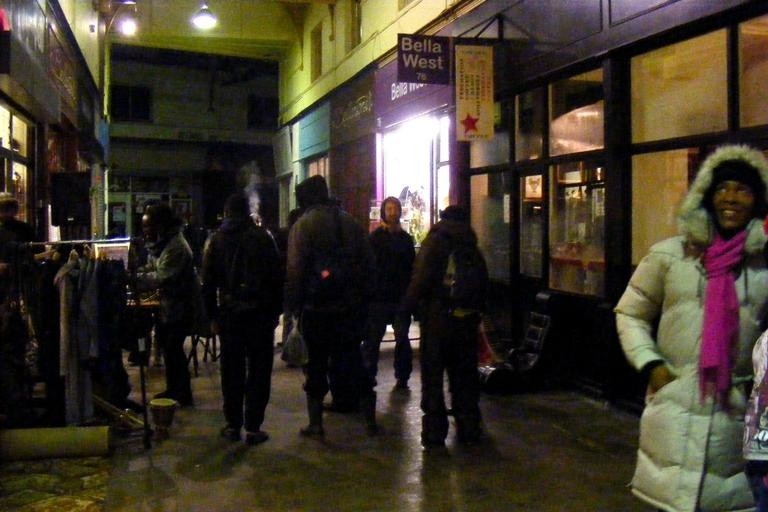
106 0 140 37
189 2 217 31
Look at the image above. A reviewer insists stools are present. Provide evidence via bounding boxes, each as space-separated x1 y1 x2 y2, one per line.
186 320 222 366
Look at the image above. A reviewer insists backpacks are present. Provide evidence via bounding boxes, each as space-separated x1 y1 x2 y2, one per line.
211 225 283 320
422 229 488 311
301 206 357 303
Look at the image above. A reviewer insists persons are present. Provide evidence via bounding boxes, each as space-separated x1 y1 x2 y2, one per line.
360 196 417 410
130 199 166 367
255 214 279 260
125 203 203 405
742 329 768 511
175 201 217 270
201 190 281 443
284 176 364 435
613 145 768 511
0 194 46 261
277 209 302 347
395 205 490 448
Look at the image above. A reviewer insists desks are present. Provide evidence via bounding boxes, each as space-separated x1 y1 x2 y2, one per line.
127 296 209 378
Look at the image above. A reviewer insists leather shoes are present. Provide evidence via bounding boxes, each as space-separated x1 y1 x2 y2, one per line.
150 392 195 410
220 424 242 443
321 401 355 415
396 375 410 393
299 424 324 436
245 430 269 446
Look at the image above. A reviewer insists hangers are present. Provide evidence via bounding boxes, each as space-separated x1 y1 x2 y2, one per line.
90 237 95 261
67 240 79 264
99 239 107 261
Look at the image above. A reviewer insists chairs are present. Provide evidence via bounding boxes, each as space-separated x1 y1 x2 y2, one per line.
474 292 557 392
